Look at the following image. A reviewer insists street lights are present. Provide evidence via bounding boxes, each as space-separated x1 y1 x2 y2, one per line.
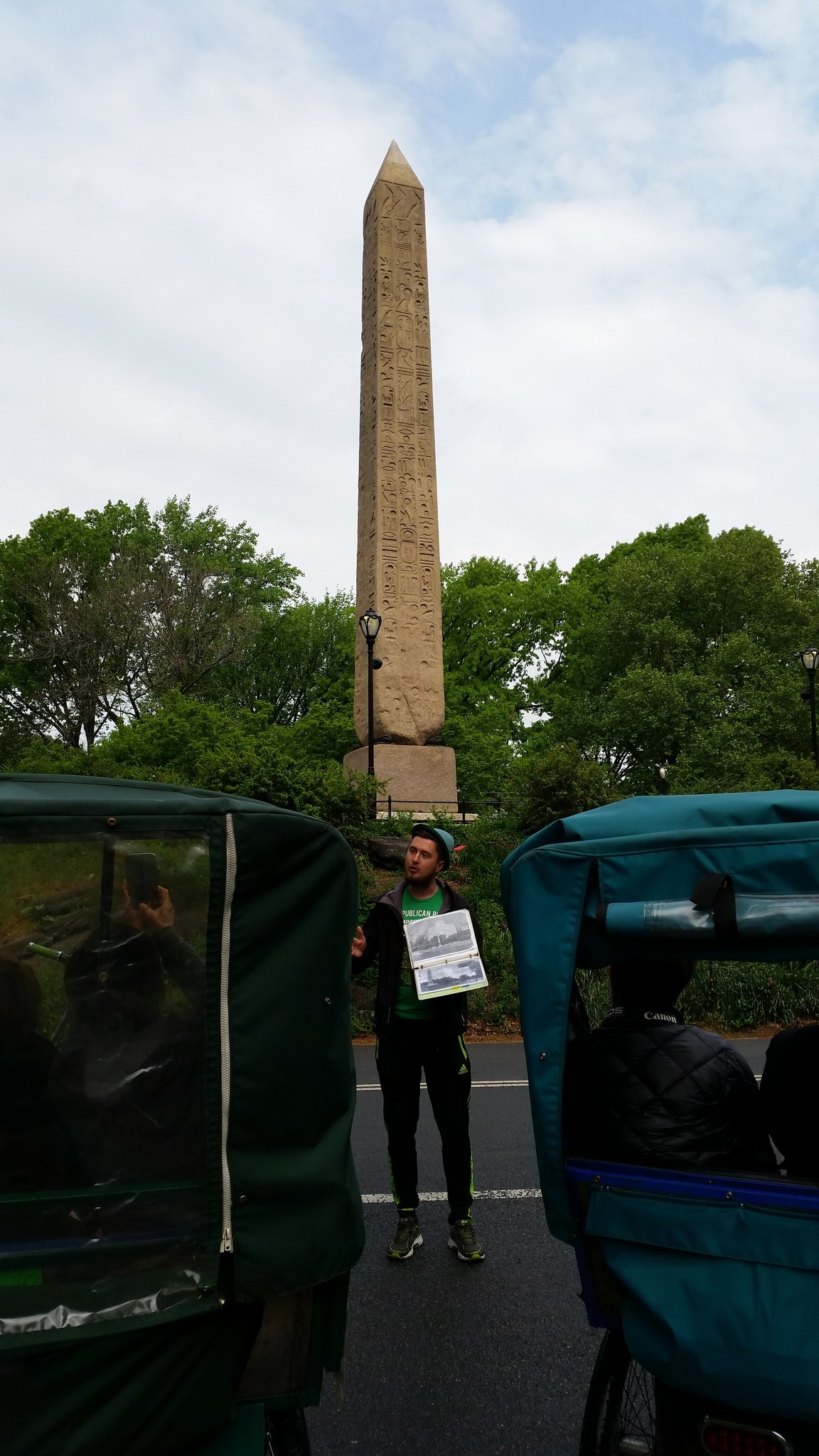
358 606 382 822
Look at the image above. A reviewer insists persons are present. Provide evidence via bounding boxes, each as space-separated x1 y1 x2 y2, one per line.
2 873 204 1196
351 824 484 1263
557 940 819 1185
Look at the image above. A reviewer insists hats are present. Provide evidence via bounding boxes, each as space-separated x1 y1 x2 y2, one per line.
412 823 453 871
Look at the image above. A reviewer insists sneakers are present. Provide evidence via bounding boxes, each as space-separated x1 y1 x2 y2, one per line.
448 1219 486 1260
386 1217 424 1258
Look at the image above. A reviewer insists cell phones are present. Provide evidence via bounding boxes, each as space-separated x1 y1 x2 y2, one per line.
125 853 160 912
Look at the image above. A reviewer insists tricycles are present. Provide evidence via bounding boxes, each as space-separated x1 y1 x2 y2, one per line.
0 767 364 1456
496 791 819 1456
